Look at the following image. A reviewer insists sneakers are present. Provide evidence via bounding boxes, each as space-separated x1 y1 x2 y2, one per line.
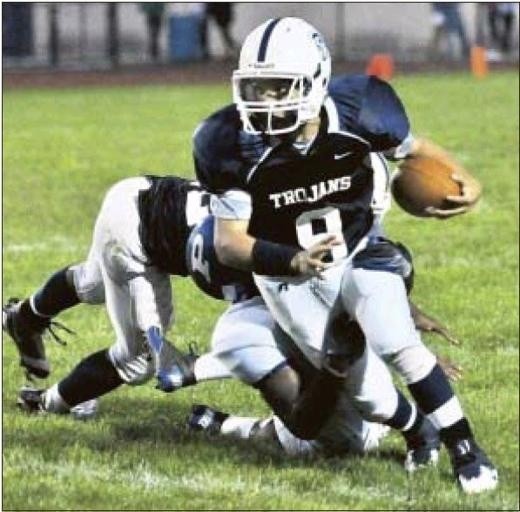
187 405 227 436
452 441 498 494
18 387 46 412
148 326 195 393
3 298 50 379
406 429 438 471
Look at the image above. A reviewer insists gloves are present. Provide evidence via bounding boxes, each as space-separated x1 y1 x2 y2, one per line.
323 311 367 374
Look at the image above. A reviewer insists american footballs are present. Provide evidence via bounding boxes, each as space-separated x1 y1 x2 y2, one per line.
389 155 461 217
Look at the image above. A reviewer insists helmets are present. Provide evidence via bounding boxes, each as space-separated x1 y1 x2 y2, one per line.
231 17 331 135
187 216 259 302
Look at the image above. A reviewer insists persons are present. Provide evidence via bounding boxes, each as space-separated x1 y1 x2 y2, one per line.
137 212 393 461
3 171 462 416
431 3 465 69
483 3 520 66
136 3 169 66
188 15 501 495
198 3 240 61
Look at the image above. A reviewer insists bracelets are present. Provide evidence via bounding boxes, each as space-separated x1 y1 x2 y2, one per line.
320 356 351 381
251 238 301 279
